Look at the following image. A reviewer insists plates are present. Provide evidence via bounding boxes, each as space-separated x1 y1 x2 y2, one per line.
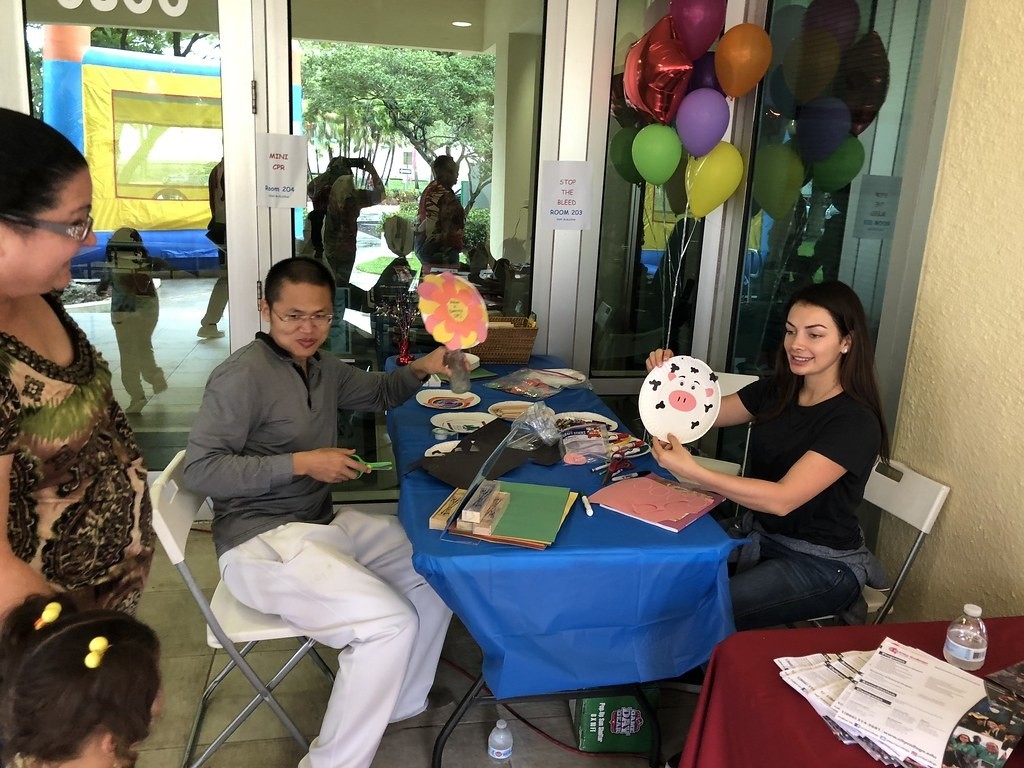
416 390 481 410
554 412 618 432
420 272 489 349
431 412 498 433
447 352 481 372
638 356 721 444
424 441 480 458
541 368 586 386
563 433 651 458
488 400 555 421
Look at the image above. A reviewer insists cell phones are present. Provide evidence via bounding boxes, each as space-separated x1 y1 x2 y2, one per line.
344 158 365 168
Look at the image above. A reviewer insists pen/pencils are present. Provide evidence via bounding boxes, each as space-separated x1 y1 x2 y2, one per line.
611 470 651 482
579 488 593 516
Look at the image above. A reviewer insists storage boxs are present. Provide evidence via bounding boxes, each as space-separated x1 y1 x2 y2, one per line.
566 684 663 754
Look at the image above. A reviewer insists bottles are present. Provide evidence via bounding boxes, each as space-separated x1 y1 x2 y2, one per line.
446 353 471 394
488 719 513 768
942 604 989 670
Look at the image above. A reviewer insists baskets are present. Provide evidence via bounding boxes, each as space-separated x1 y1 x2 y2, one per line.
466 316 539 365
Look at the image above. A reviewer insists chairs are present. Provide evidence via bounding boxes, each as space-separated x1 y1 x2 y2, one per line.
805 454 951 626
146 447 334 767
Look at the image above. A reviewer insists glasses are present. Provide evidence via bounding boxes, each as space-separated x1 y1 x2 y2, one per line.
269 304 333 326
0 213 93 242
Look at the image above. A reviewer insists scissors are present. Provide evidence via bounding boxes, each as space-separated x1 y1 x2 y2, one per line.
602 451 632 484
347 455 394 479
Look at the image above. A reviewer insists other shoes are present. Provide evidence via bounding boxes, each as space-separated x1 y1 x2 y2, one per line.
647 665 705 693
196 326 225 338
126 392 149 415
664 750 686 768
151 367 168 394
422 686 454 712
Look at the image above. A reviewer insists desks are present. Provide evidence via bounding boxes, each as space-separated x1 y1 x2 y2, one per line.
676 615 1023 768
382 351 749 767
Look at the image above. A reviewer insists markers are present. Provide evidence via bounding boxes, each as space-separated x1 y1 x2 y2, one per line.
608 433 649 457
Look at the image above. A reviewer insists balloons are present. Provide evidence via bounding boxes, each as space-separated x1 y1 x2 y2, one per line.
752 0 890 222
607 0 690 216
622 0 771 217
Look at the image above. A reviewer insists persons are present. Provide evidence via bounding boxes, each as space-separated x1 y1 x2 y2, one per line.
942 690 1024 768
414 156 466 270
184 256 472 768
307 156 386 290
193 139 228 336
644 278 888 696
96 228 171 416
0 592 163 768
0 106 154 618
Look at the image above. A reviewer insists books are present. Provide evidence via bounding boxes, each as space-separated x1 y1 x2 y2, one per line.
600 471 726 533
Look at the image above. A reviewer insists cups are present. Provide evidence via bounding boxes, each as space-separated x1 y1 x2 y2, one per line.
431 426 448 440
